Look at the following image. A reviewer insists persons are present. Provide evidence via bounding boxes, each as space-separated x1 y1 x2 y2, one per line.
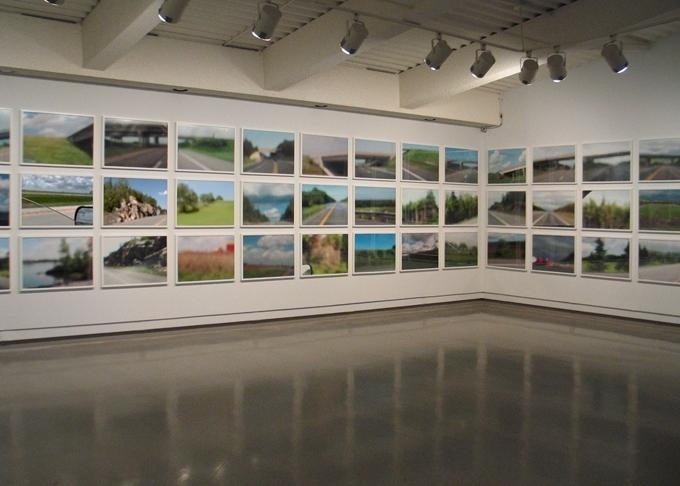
115 195 161 223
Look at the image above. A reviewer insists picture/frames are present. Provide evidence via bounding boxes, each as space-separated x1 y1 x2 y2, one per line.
398 140 440 184
0 109 236 292
531 188 577 230
531 233 578 276
579 236 633 282
351 184 397 230
239 180 298 229
485 189 528 229
580 189 634 233
239 126 297 177
532 144 578 185
298 231 349 279
638 136 680 183
484 230 528 273
298 132 350 180
298 181 349 229
441 231 481 269
352 136 396 182
398 231 440 273
485 146 530 187
442 146 481 187
352 232 397 275
240 231 296 281
442 188 481 227
578 140 634 184
636 188 680 234
636 237 680 286
398 186 440 228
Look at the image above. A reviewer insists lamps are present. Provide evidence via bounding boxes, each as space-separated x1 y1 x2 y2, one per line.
469 43 496 79
158 1 191 25
518 50 538 84
424 33 451 72
251 2 284 42
546 49 567 84
338 14 369 56
602 37 629 75
